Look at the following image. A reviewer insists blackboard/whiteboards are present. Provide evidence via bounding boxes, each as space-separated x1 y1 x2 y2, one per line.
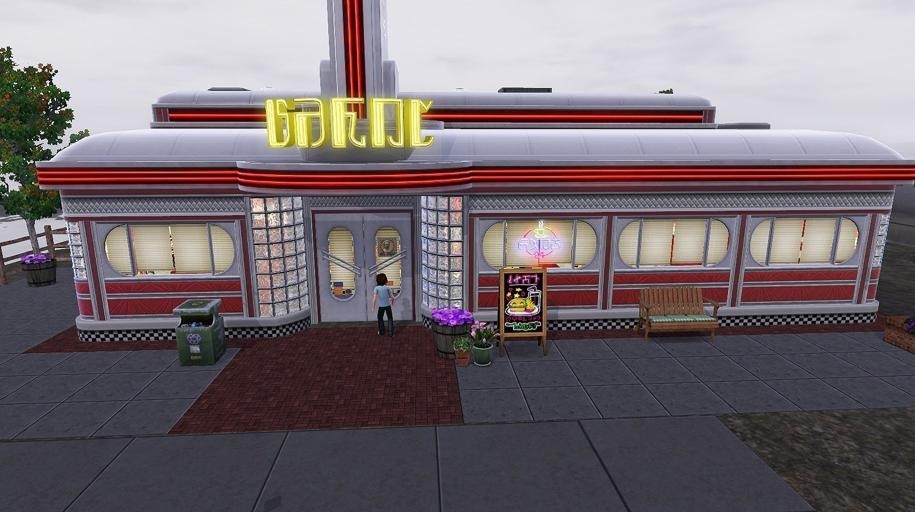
499 269 547 337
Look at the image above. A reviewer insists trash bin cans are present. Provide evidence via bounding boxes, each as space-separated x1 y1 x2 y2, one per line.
172 299 226 365
